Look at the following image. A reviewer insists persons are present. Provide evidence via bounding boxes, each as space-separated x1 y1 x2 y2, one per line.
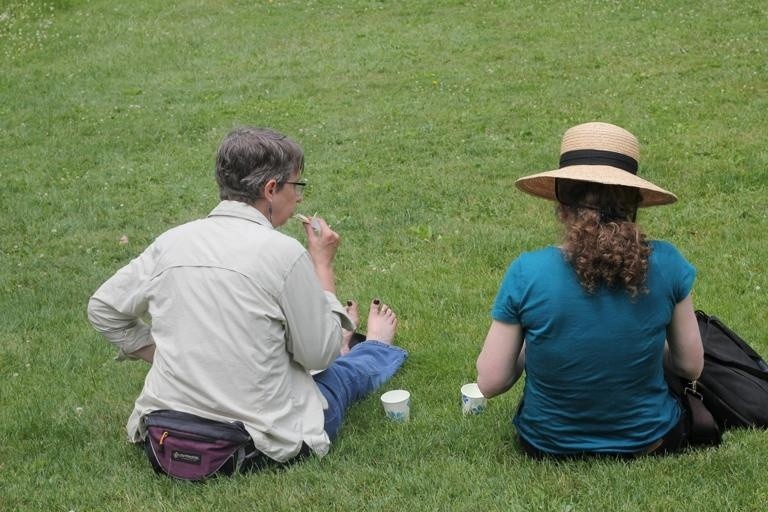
474 118 706 459
85 124 407 477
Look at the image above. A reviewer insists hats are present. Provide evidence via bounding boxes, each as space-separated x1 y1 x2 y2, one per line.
515 121 678 205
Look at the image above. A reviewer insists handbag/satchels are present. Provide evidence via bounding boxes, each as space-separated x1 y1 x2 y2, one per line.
145 409 254 482
666 310 768 432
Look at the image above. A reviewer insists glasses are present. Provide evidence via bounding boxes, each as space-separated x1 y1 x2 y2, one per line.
283 176 307 194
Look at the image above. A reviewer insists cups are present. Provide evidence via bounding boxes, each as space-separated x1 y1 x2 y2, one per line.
460 383 488 416
381 389 411 420
